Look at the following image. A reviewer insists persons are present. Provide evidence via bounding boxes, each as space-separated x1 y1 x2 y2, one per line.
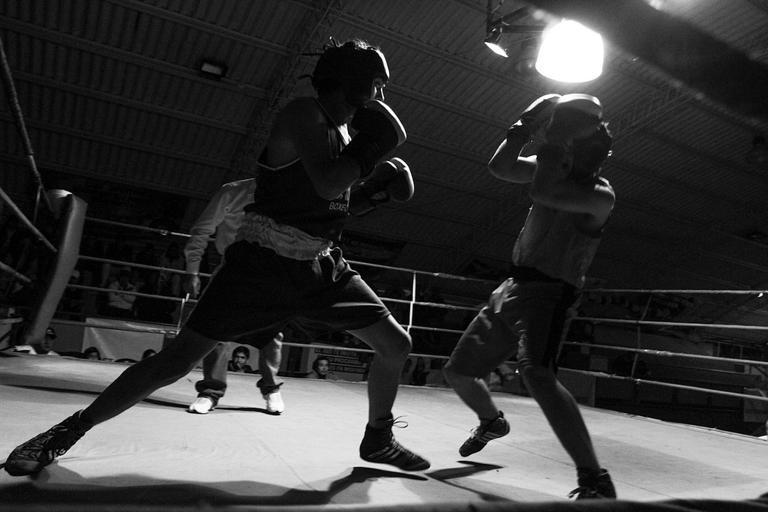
5 39 430 476
443 92 617 502
182 178 284 416
1 247 430 384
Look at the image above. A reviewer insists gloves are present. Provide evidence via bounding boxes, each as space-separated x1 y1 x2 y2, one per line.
536 93 602 168
339 98 406 176
348 157 414 218
507 93 561 143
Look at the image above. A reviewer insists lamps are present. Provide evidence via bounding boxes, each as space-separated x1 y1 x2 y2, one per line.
481 28 511 59
196 63 228 81
511 41 538 74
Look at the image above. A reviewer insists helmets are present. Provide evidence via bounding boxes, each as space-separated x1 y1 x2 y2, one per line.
561 124 613 185
310 40 389 107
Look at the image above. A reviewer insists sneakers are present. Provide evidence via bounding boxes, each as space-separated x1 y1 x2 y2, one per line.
5 409 84 477
360 424 429 470
459 410 510 457
262 390 284 414
569 468 616 500
189 396 215 414
